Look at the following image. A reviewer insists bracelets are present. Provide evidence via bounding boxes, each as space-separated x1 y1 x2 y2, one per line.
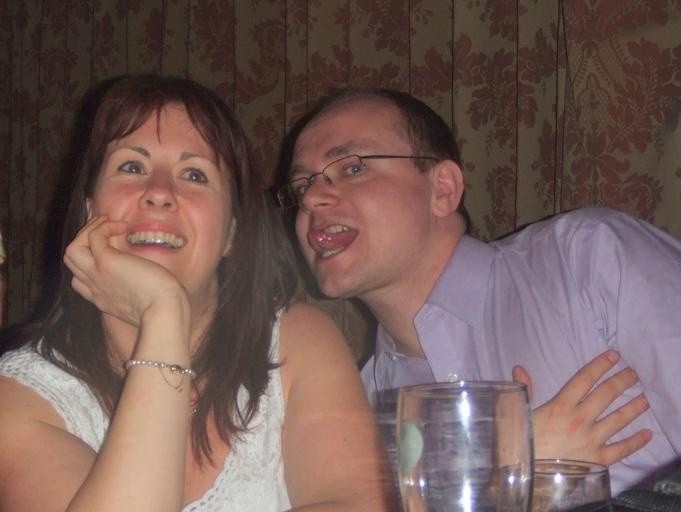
123 359 198 388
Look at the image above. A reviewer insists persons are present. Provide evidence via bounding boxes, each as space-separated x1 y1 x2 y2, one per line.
0 70 404 512
287 87 681 512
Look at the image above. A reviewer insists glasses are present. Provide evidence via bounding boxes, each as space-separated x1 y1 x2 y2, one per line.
276 155 442 211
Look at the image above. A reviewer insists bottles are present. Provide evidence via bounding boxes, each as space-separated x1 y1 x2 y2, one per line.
396 380 611 512
